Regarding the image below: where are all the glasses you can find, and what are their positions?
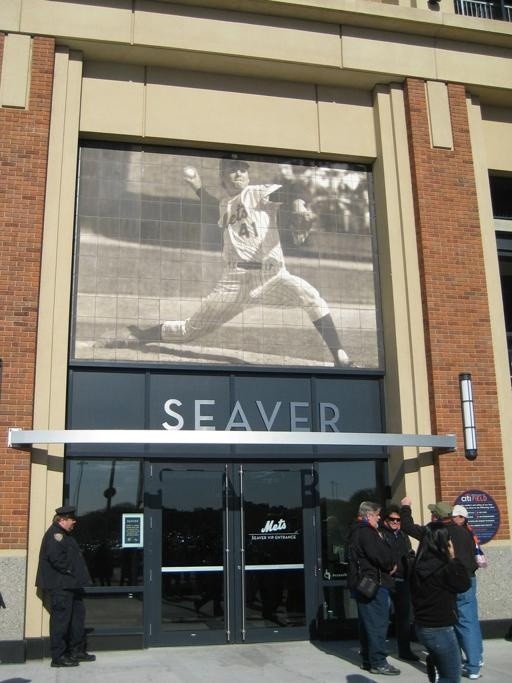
[387,516,401,522]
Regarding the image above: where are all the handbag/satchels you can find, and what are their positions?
[354,560,379,602]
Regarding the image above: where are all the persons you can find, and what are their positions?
[34,505,96,667]
[269,158,371,257]
[126,157,358,367]
[321,495,489,683]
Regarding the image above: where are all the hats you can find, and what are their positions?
[55,506,78,514]
[219,156,249,169]
[427,502,452,519]
[450,504,469,520]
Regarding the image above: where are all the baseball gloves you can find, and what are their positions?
[289,210,317,245]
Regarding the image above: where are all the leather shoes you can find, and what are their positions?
[50,656,79,667]
[400,653,420,662]
[70,651,96,662]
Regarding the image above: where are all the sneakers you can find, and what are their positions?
[360,661,371,670]
[369,662,400,675]
[98,324,140,349]
[461,657,484,667]
[469,665,481,679]
[333,349,359,368]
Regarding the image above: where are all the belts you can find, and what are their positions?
[236,262,267,270]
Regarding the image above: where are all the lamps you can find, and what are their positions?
[459,370,480,460]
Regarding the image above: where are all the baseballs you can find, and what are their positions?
[183,167,196,178]
